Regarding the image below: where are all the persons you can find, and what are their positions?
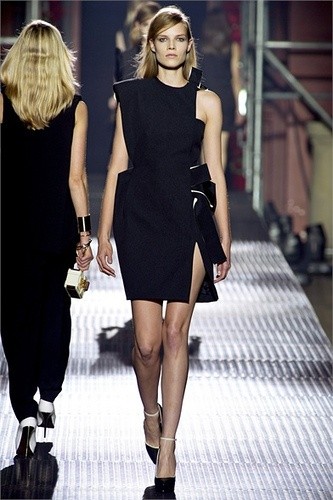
[94,6,231,493]
[110,0,244,181]
[0,20,93,464]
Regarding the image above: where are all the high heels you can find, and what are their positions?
[14,416,39,457]
[144,403,177,496]
[38,399,55,438]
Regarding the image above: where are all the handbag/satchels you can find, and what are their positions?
[63,238,92,299]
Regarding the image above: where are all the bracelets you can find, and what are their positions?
[76,214,92,250]
